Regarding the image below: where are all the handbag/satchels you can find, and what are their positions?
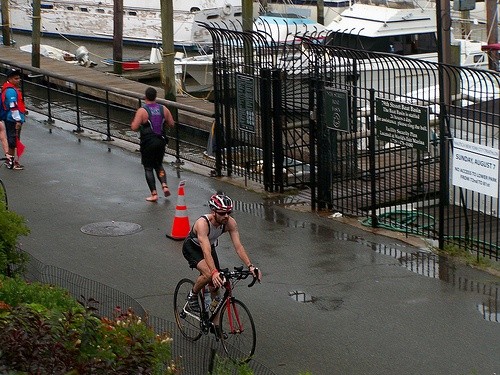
[0,92,9,121]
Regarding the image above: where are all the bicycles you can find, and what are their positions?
[0,156,14,211]
[173,266,261,364]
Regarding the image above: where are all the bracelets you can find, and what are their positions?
[211,269,218,276]
[5,152,10,156]
[248,264,254,269]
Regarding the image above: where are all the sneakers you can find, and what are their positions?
[185,290,201,315]
[210,326,228,340]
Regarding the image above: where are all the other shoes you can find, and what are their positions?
[4,160,24,170]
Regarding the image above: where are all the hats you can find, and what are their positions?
[7,68,20,79]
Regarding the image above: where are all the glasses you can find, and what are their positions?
[213,210,232,216]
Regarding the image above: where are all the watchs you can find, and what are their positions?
[181,192,260,332]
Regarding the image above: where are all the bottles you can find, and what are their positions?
[210,295,220,311]
[204,289,211,311]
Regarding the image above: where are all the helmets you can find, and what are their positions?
[208,193,233,212]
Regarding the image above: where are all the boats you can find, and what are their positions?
[7,0,500,150]
[0,33,16,48]
[101,58,163,72]
[203,146,319,192]
[19,43,98,90]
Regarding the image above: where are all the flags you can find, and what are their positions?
[15,136,25,158]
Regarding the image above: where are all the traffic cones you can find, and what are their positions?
[166,183,191,240]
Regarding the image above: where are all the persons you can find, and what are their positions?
[0,120,11,163]
[5,68,28,170]
[132,87,176,201]
